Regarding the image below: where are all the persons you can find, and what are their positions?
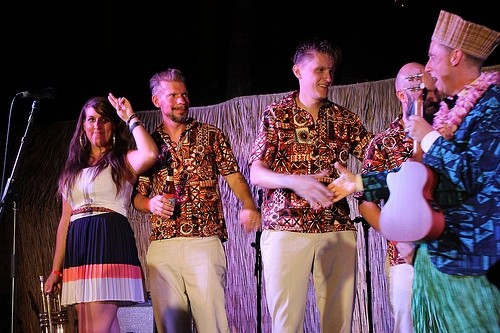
[44,93,158,333]
[131,70,261,333]
[327,10,500,333]
[249,40,376,333]
[357,63,438,333]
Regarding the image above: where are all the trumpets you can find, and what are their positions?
[39,274,53,332]
[52,288,69,333]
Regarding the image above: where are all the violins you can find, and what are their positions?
[377,83,445,242]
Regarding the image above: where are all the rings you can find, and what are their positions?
[251,221,256,224]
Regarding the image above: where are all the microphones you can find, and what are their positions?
[16,87,55,99]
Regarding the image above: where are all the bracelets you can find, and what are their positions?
[127,113,145,133]
[51,270,61,275]
[145,197,153,212]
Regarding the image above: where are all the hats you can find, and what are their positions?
[432,9,499,60]
